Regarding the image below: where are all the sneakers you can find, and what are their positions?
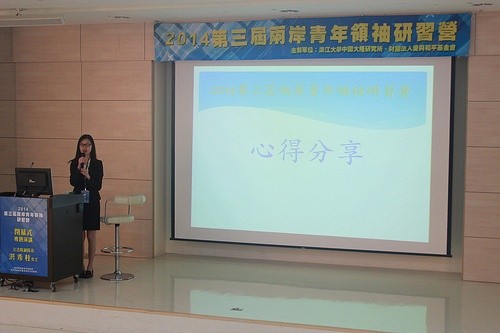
[79,270,86,278]
[85,270,93,278]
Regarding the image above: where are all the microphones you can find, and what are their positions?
[81,153,85,168]
[30,162,34,167]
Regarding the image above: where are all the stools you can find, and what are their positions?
[99,195,146,282]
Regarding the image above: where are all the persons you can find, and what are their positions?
[69,134,104,279]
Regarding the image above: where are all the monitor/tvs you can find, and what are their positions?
[15,167,52,198]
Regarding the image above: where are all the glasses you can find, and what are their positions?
[79,143,92,148]
